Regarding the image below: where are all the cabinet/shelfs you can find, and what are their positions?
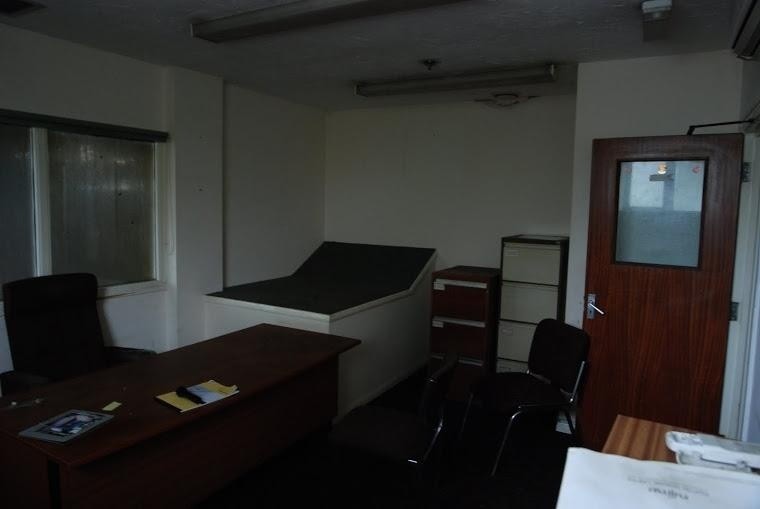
[496,232,567,377]
[430,263,498,377]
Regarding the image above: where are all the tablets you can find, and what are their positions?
[18,407,116,447]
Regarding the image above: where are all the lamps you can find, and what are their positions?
[354,63,558,98]
[188,0,451,46]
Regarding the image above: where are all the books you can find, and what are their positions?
[155,379,241,414]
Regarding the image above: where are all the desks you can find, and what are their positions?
[2,324,366,508]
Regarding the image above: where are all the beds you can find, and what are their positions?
[203,241,442,425]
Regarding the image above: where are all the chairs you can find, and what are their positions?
[457,318,590,475]
[3,272,159,387]
